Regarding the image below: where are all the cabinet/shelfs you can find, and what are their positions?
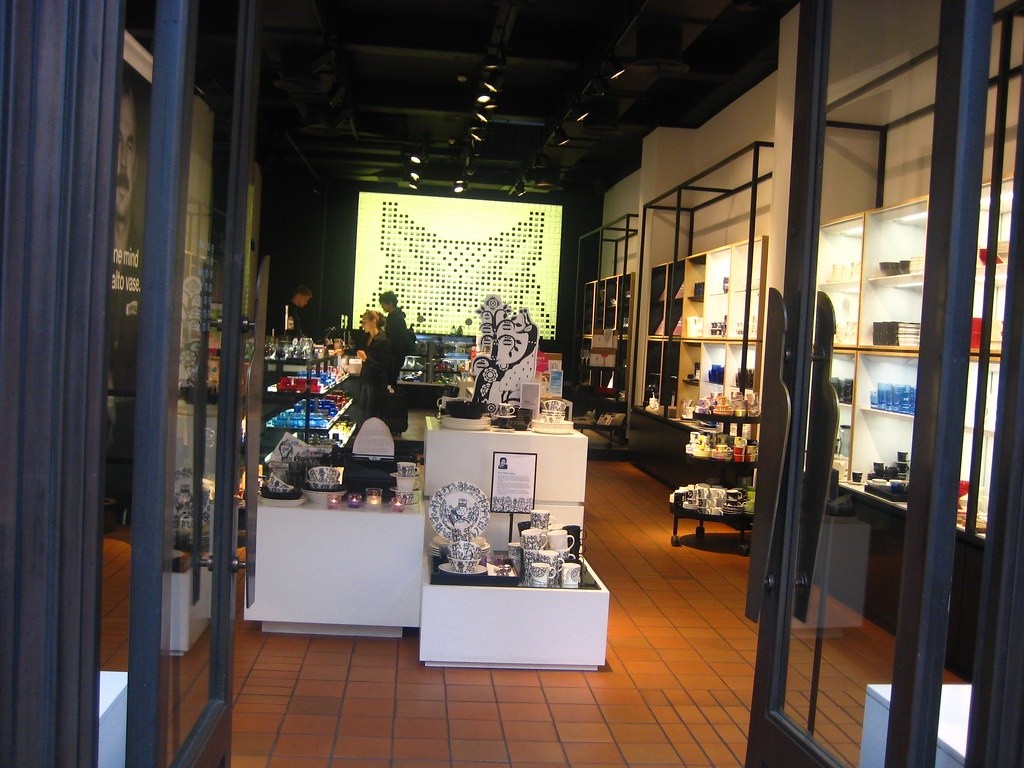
[813,176,1015,538]
[641,231,769,442]
[671,411,762,555]
[581,271,636,399]
[398,335,473,386]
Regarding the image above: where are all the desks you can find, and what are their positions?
[423,416,589,554]
[244,490,424,638]
[264,348,356,450]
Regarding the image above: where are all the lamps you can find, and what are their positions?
[409,43,625,200]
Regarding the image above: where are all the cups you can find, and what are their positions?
[498,403,515,416]
[326,462,421,511]
[820,262,862,484]
[868,382,916,414]
[434,385,461,422]
[645,276,761,427]
[685,431,758,461]
[265,337,353,447]
[668,483,754,515]
[507,508,587,588]
[853,451,909,502]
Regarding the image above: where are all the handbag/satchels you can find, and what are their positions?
[366,355,400,382]
[401,324,418,356]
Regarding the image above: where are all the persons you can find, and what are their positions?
[379,291,407,385]
[284,285,312,337]
[114,87,139,338]
[357,311,387,418]
[498,457,507,469]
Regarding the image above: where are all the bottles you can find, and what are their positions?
[450,325,456,336]
[456,326,463,336]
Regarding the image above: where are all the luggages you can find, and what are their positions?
[383,383,409,437]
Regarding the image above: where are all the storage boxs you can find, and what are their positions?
[419,545,610,670]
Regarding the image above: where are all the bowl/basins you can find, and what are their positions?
[541,400,566,422]
[510,408,532,430]
[447,542,482,559]
[267,487,294,493]
[447,554,481,572]
[300,467,347,502]
[268,473,291,487]
[445,400,487,418]
[871,254,925,349]
[970,241,1011,353]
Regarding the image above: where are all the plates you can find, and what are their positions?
[442,414,492,431]
[427,482,491,557]
[257,487,306,506]
[495,415,516,418]
[531,419,574,435]
[438,563,488,575]
